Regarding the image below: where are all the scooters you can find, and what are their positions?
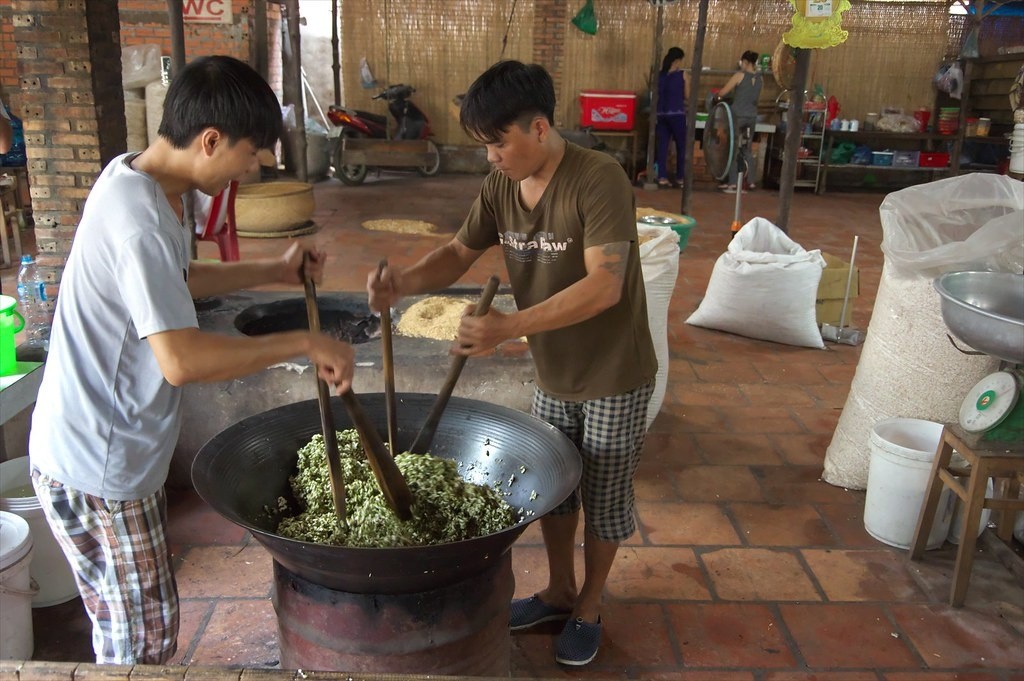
[328,83,444,185]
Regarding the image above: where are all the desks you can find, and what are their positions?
[767,90,828,194]
[588,131,639,185]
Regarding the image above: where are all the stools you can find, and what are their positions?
[0,186,23,265]
[912,422,1024,607]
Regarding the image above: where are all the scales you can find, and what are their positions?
[933,270,1024,459]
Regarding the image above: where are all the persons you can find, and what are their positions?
[29,56,353,665]
[715,51,764,192]
[0,98,12,154]
[656,47,690,187]
[366,60,658,666]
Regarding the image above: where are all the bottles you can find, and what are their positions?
[865,111,879,131]
[965,117,992,137]
[913,106,932,134]
[17,256,51,350]
[829,117,859,131]
[0,105,27,166]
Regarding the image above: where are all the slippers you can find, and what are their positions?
[510,593,570,630]
[556,612,602,666]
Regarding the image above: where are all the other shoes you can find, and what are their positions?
[719,184,756,193]
[659,179,673,188]
[677,180,684,188]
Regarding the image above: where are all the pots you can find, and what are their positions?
[192,393,583,595]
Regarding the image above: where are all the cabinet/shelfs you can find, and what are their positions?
[820,127,961,194]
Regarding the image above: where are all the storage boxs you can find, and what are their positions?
[890,149,921,167]
[580,90,638,132]
[920,152,950,167]
[872,152,892,166]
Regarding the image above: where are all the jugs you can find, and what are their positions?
[824,95,842,127]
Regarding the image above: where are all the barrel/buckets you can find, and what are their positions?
[1009,124,1024,174]
[0,456,80,608]
[0,511,40,662]
[865,418,960,549]
[1,296,25,376]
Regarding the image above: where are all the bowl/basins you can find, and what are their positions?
[640,215,676,225]
[934,269,1024,365]
[939,108,959,134]
[654,214,696,252]
